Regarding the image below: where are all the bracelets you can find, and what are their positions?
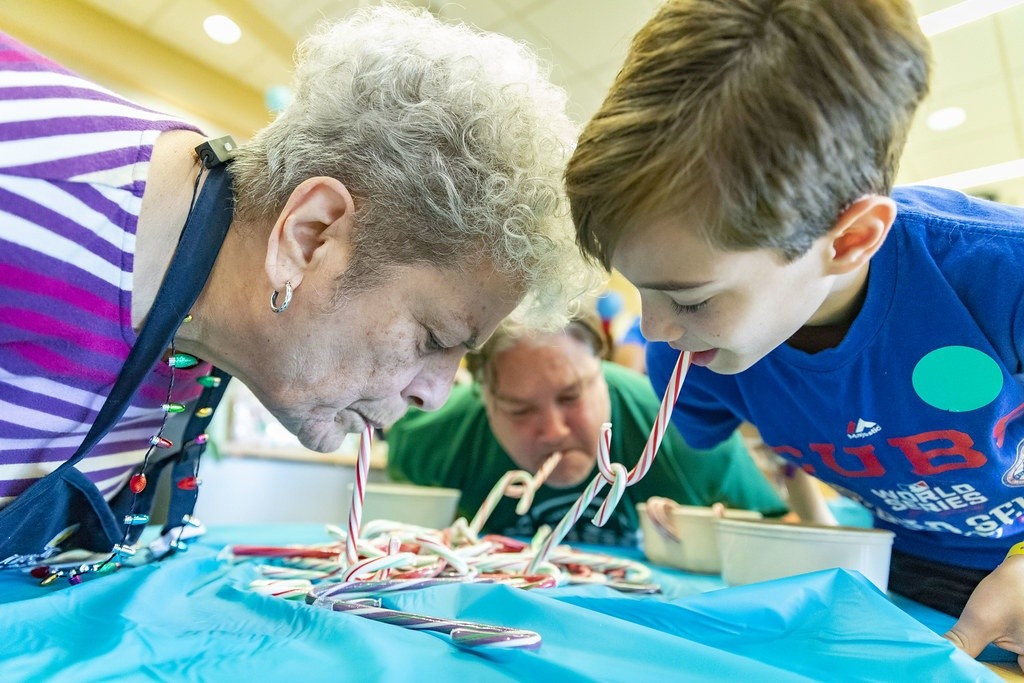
[1006,542,1024,557]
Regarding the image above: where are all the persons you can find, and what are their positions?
[385,304,789,549]
[0,7,607,553]
[564,0,1024,678]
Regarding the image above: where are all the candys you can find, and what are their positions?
[233,350,692,652]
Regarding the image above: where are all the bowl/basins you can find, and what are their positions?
[637,503,762,575]
[711,517,898,595]
[346,481,459,531]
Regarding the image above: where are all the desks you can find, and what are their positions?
[192,445,387,520]
[0,520,1024,683]
